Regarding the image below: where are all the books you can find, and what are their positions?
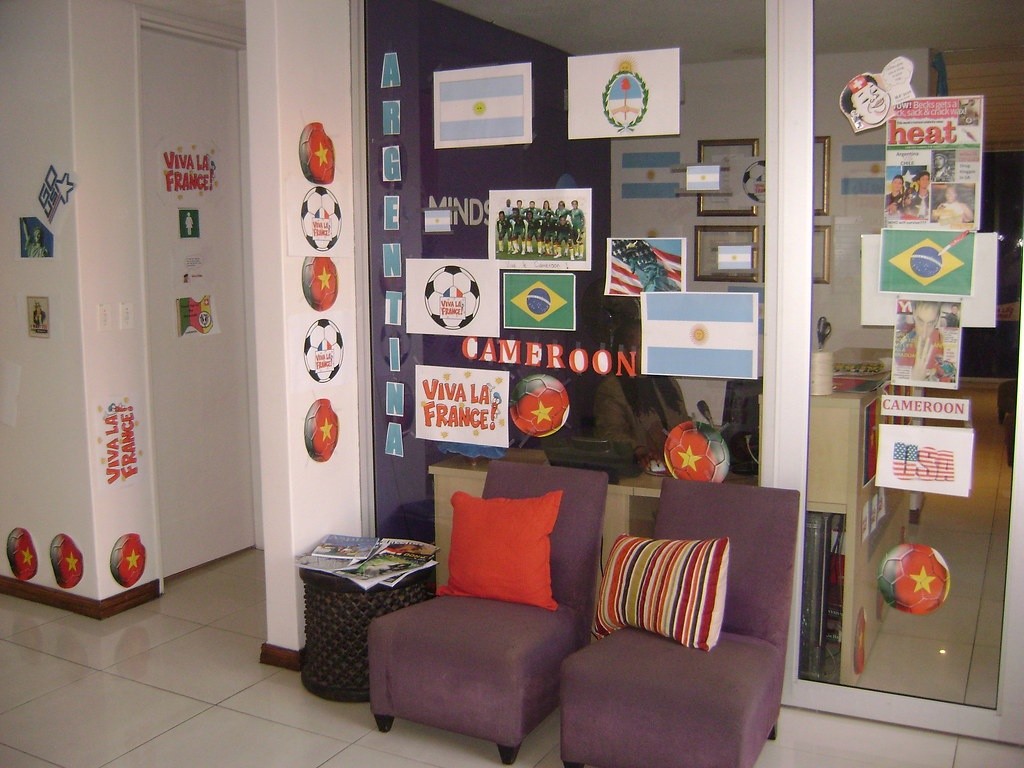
[298,534,440,589]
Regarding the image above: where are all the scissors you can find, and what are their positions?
[816,315,832,352]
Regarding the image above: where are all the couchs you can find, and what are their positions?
[368,462,609,765]
[559,479,801,768]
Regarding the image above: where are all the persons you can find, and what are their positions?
[946,305,959,328]
[886,174,903,217]
[895,300,944,382]
[497,199,586,260]
[905,171,930,220]
[934,185,974,224]
[592,320,688,476]
[627,251,681,292]
[933,150,952,182]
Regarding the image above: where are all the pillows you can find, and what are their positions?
[593,531,735,652]
[437,488,566,609]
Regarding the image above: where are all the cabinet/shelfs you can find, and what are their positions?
[757,345,929,685]
[428,447,758,641]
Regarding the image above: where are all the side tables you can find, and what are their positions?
[298,560,429,705]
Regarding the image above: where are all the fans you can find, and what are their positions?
[581,278,641,351]
